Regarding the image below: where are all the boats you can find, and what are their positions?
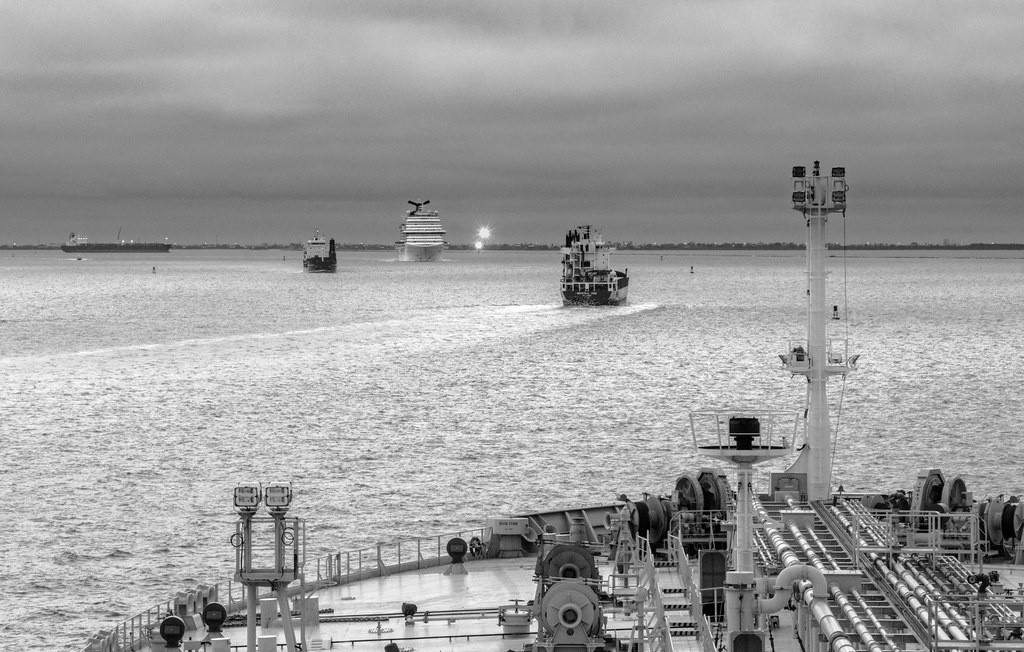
[558,223,629,307]
[301,229,337,274]
[395,198,449,262]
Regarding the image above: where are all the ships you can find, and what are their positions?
[59,227,170,254]
